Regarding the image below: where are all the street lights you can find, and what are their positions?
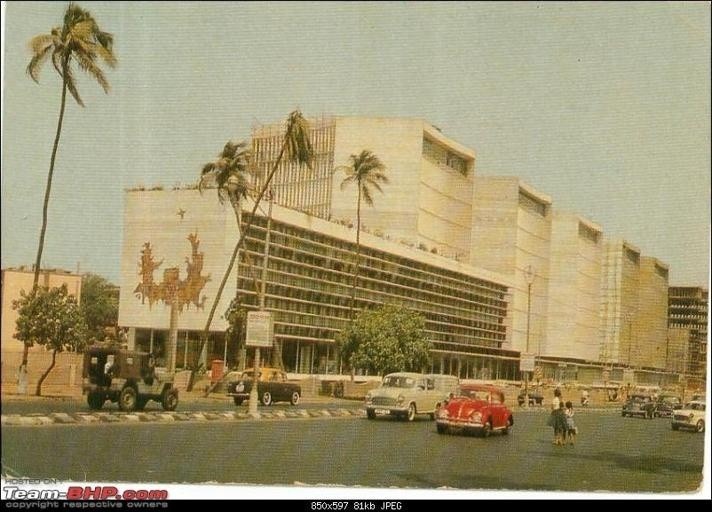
[522,264,536,409]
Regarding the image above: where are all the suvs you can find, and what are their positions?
[82,347,179,412]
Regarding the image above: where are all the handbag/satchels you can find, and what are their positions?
[573,426,577,434]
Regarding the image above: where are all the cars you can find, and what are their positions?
[518,391,545,408]
[364,372,459,422]
[621,394,706,434]
[436,383,514,438]
[226,367,301,406]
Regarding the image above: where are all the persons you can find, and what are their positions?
[547,389,579,447]
